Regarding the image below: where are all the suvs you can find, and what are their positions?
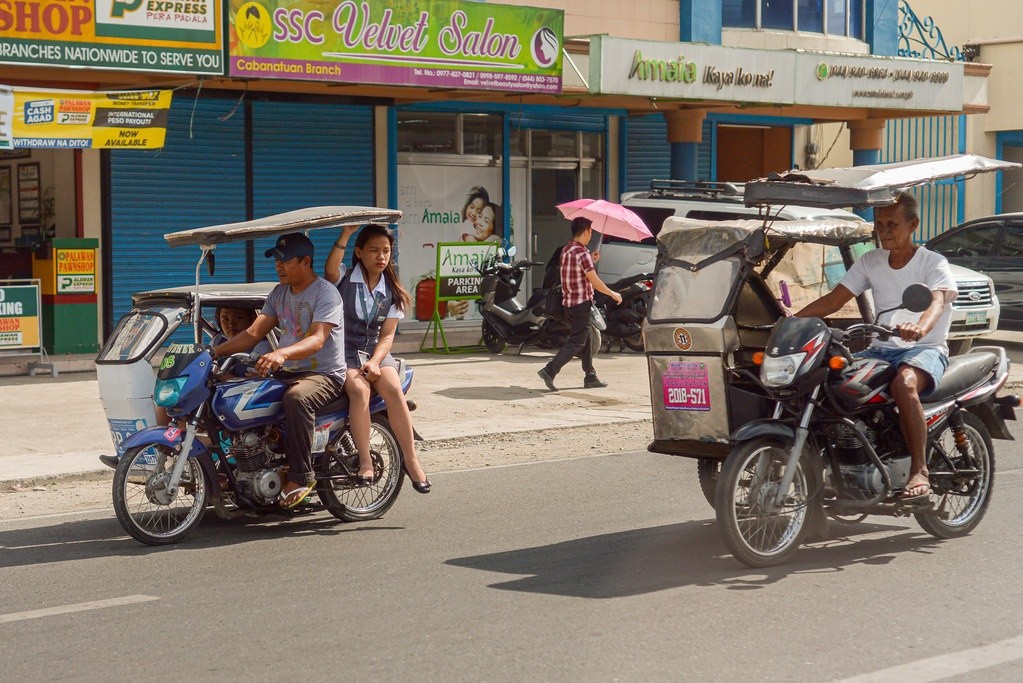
[593,177,1001,359]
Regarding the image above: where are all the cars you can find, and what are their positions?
[918,211,1023,335]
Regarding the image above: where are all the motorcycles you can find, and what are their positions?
[641,152,1023,569]
[542,245,654,353]
[468,236,609,359]
[97,205,413,546]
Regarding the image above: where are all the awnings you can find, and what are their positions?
[164,205,404,247]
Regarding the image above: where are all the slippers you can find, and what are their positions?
[900,482,932,502]
[279,478,318,510]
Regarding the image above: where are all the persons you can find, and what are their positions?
[99,307,271,470]
[537,217,622,392]
[210,233,347,509]
[325,225,432,493]
[447,185,501,317]
[535,246,566,348]
[788,192,960,504]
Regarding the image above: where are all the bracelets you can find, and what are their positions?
[333,241,347,250]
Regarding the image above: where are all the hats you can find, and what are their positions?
[265,232,315,260]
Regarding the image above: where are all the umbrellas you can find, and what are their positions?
[555,198,655,252]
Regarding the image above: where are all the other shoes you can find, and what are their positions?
[538,369,558,391]
[584,380,608,388]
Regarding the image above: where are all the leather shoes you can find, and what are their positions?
[401,459,432,494]
[356,467,375,484]
